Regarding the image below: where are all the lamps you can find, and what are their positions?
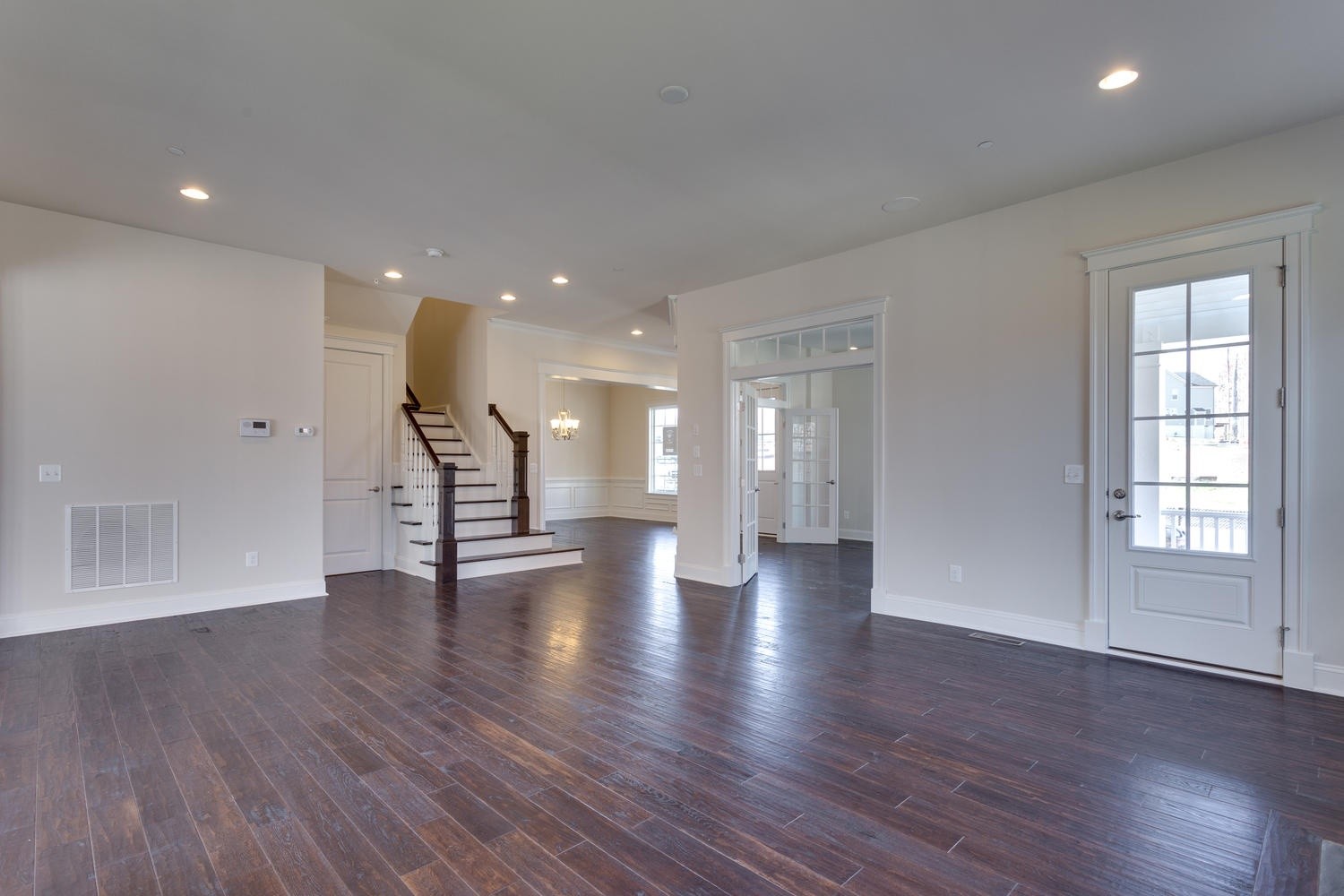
[548,378,579,441]
